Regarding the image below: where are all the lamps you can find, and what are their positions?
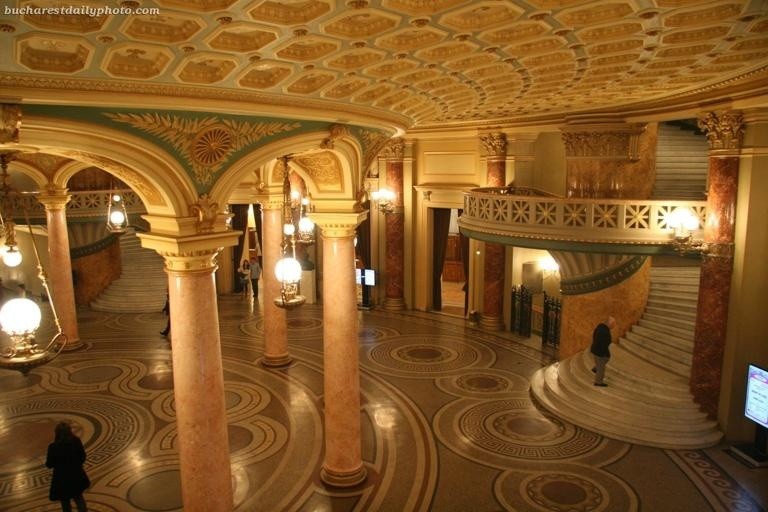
[668,205,700,242]
[368,186,396,217]
[282,182,317,243]
[105,178,131,234]
[1,212,25,266]
[272,211,308,309]
[0,245,67,369]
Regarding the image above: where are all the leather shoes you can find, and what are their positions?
[592,368,596,373]
[160,330,168,336]
[594,382,608,387]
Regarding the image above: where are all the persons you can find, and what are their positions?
[238,258,250,294]
[159,294,170,336]
[588,316,616,387]
[250,256,260,297]
[45,422,92,511]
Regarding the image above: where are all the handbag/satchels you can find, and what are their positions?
[239,272,247,278]
